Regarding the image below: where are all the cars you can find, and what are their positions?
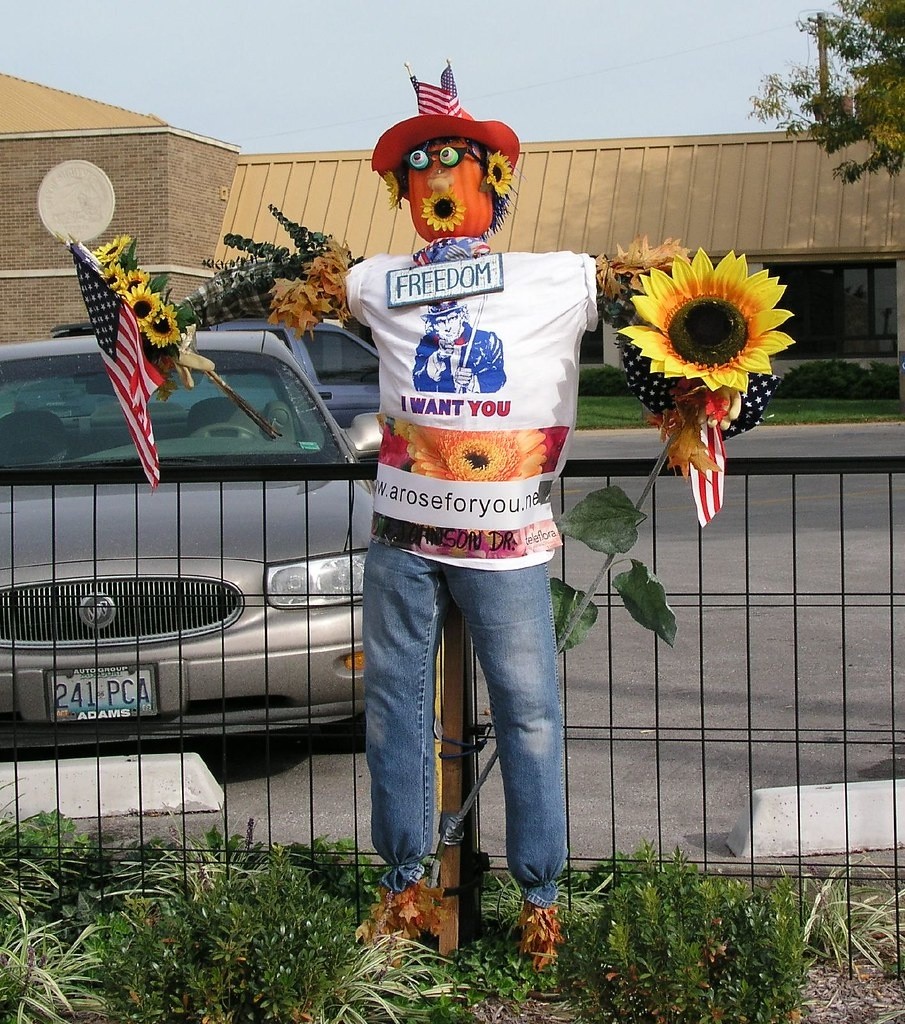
[198,317,381,461]
[13,377,96,423]
[1,327,376,751]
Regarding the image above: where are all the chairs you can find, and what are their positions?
[0,410,68,466]
[186,396,262,437]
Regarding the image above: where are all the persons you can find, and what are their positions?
[49,59,797,972]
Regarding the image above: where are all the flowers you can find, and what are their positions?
[384,169,403,216]
[613,249,796,484]
[421,188,466,234]
[482,146,511,200]
[369,417,566,558]
[88,235,186,356]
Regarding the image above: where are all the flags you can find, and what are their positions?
[64,239,165,491]
[405,65,475,121]
[621,335,784,529]
